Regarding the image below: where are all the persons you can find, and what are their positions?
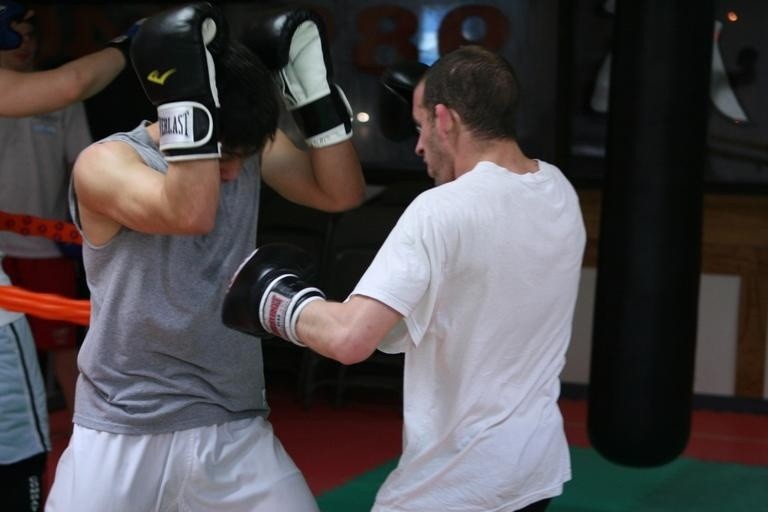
[2,18,146,512]
[8,5,92,434]
[219,44,591,512]
[40,2,366,512]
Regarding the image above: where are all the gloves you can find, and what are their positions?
[255,6,357,149]
[219,238,328,348]
[126,0,234,162]
